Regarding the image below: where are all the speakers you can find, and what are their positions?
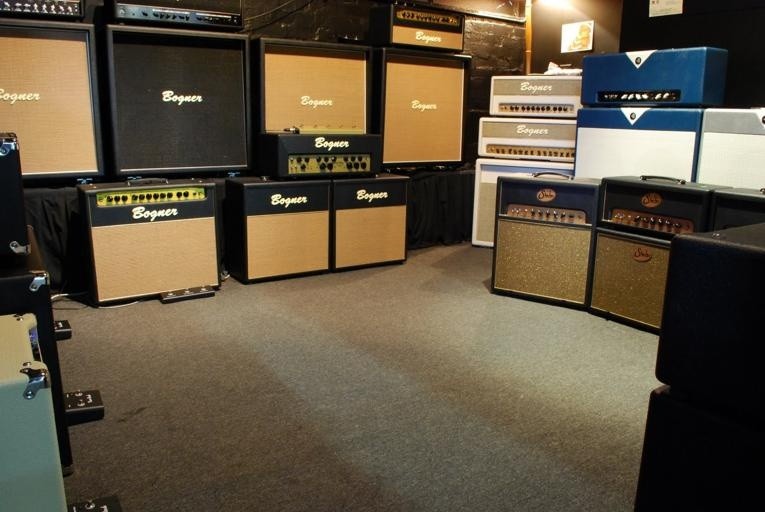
[378,50,471,165]
[633,221,764,510]
[106,23,252,175]
[372,2,466,54]
[225,178,330,283]
[257,37,371,134]
[472,46,765,337]
[332,173,413,273]
[0,18,104,179]
[77,178,220,302]
